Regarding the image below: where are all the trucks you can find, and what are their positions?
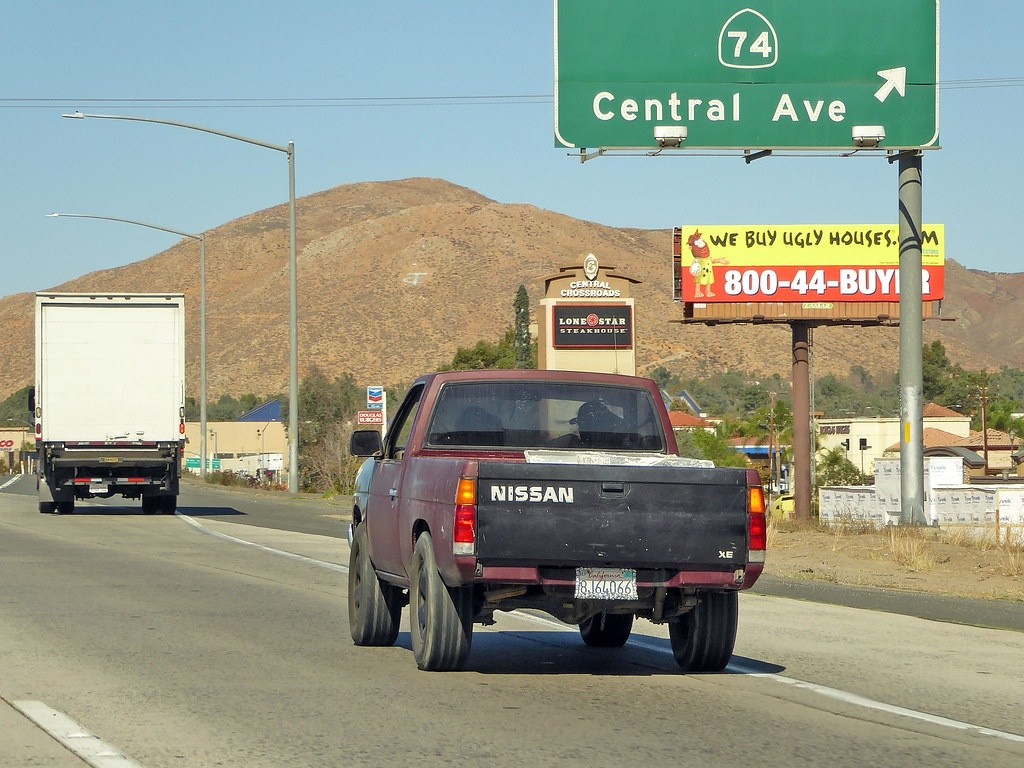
[27,290,188,516]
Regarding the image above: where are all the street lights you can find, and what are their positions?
[62,111,298,494]
[1010,430,1016,475]
[45,213,206,481]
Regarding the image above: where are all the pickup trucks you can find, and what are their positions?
[346,369,767,675]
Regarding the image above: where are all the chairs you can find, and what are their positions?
[578,413,629,449]
[451,414,505,446]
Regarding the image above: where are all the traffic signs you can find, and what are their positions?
[552,0,945,151]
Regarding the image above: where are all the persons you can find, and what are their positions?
[455,406,494,434]
[569,400,613,450]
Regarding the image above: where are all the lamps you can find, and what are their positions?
[654,126,688,147]
[851,126,885,147]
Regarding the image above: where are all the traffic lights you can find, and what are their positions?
[268,471,273,480]
[256,468,260,479]
[841,439,849,450]
